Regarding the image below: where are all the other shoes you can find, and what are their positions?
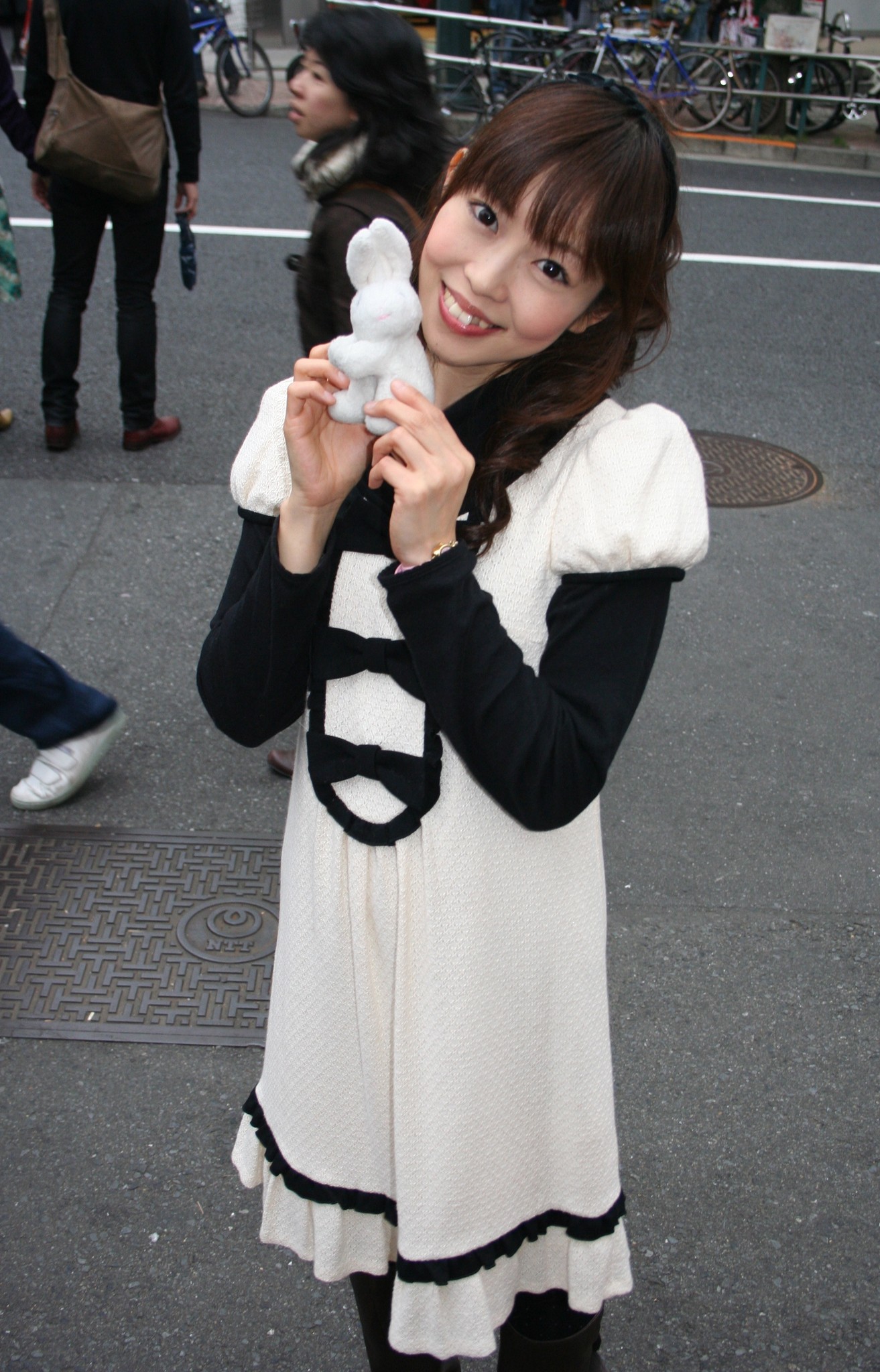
[268,748,296,779]
[0,408,12,428]
[196,87,206,99]
[227,73,239,95]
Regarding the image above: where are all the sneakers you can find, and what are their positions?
[9,708,127,810]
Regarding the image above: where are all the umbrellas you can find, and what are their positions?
[174,210,197,290]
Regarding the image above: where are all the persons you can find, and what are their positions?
[0,620,130,811]
[196,80,708,1372]
[0,0,240,453]
[268,5,451,780]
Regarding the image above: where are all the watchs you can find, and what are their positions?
[393,541,458,574]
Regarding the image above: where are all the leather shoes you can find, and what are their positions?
[123,416,179,450]
[46,418,80,451]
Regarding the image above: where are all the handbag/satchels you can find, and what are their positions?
[718,0,757,66]
[34,73,166,195]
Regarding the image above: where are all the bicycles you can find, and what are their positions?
[191,0,274,118]
[424,0,880,151]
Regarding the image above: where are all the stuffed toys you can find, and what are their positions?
[326,219,435,436]
